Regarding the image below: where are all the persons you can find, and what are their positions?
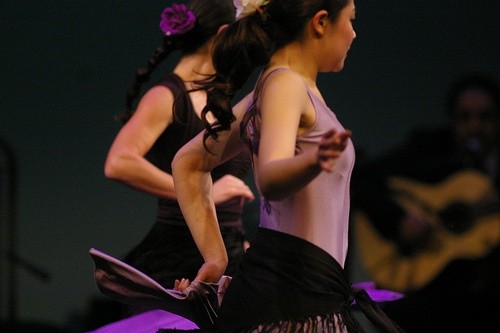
[105,1,499,333]
